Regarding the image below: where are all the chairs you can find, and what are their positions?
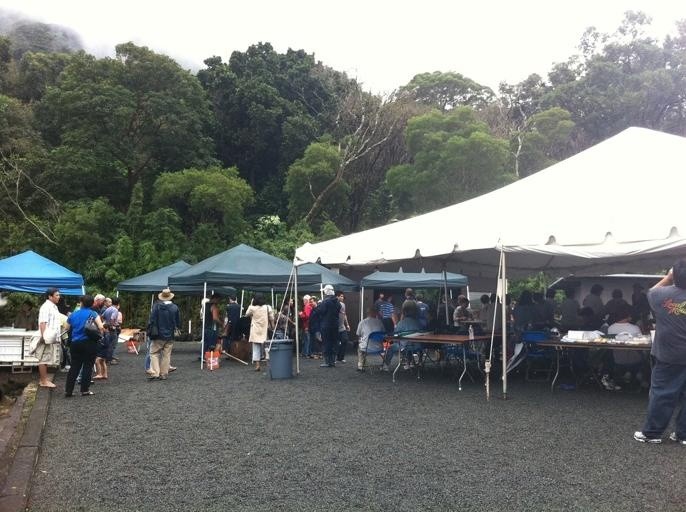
[358,319,656,392]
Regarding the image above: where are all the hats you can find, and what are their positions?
[324,285,335,296]
[158,288,175,301]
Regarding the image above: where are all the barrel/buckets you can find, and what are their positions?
[127,340,138,354]
[204,350,220,371]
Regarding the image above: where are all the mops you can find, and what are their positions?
[207,344,215,371]
[213,343,249,365]
[129,337,139,355]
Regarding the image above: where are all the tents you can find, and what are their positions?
[0,244,468,369]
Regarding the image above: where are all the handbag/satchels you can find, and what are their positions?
[85,317,105,344]
[149,320,159,340]
[173,327,182,337]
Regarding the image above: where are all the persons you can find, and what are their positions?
[14,255,685,396]
[633,259,686,446]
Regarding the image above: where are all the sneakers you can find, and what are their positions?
[669,431,686,445]
[147,365,176,380]
[633,431,662,443]
[601,374,622,390]
